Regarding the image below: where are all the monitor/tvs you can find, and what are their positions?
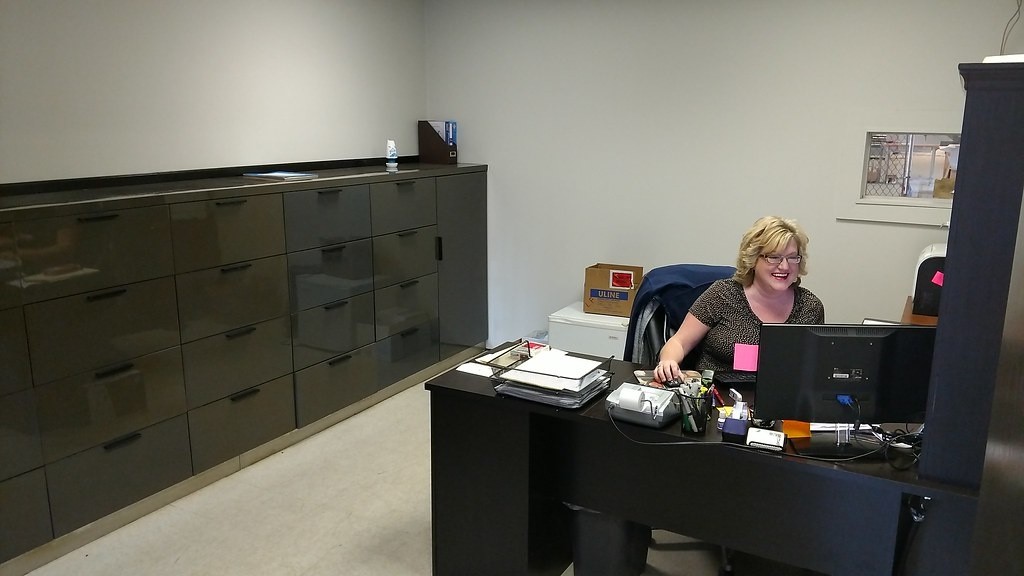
[752,323,936,460]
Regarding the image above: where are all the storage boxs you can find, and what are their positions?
[583,262,644,317]
[547,302,629,360]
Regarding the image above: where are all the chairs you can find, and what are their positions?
[622,264,737,365]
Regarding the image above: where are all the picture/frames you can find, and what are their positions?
[835,107,964,228]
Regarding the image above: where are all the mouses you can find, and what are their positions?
[663,373,680,387]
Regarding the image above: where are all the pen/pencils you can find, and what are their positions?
[713,389,725,405]
[678,381,714,435]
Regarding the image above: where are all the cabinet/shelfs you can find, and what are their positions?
[0,162,488,576]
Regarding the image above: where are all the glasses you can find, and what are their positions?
[758,254,802,264]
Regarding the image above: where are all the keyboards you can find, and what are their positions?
[714,372,757,385]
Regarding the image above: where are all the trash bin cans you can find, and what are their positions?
[566,503,653,576]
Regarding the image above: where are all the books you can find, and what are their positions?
[244,170,320,181]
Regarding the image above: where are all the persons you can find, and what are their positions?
[653,218,825,383]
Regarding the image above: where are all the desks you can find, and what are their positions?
[425,341,979,576]
